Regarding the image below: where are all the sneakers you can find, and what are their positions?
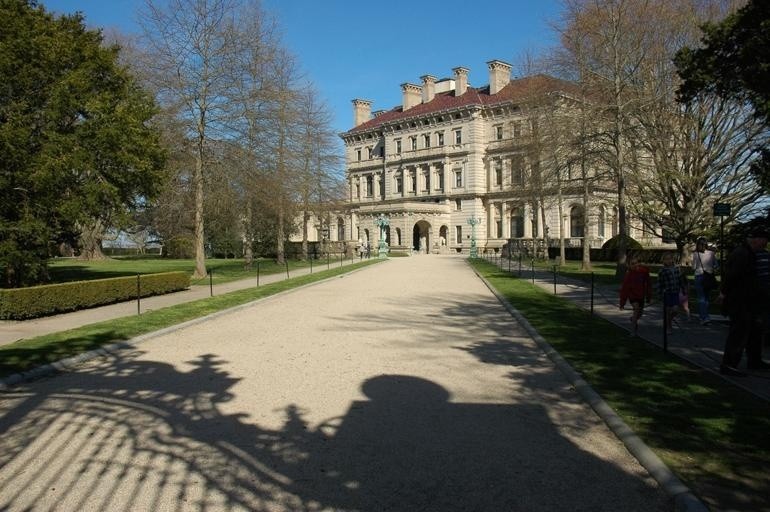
[747,360,770,372]
[700,319,713,327]
[719,364,747,377]
[630,317,639,329]
[632,327,640,338]
[666,328,673,335]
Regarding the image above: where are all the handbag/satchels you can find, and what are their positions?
[701,269,718,291]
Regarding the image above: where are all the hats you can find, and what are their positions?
[747,229,770,238]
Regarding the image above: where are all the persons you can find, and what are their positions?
[619,256,654,336]
[692,236,719,323]
[716,227,769,376]
[757,248,769,364]
[359,243,364,259]
[365,243,370,258]
[679,283,694,322]
[657,253,690,334]
